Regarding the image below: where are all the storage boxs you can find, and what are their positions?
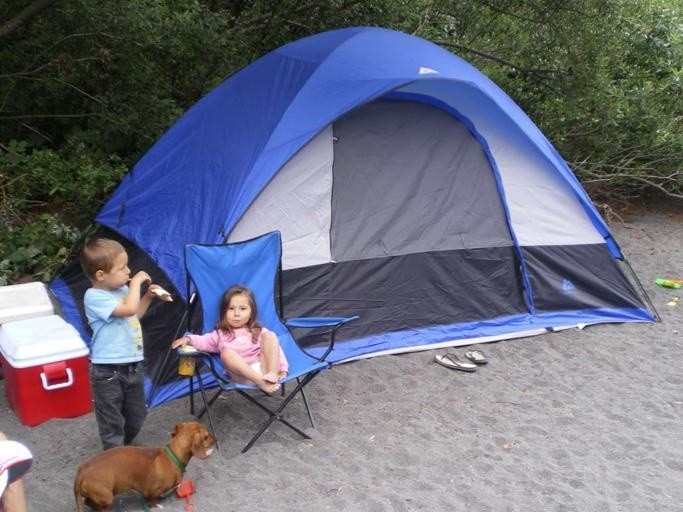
[1,281,94,429]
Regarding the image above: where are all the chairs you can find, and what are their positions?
[170,230,361,456]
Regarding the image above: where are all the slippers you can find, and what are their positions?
[434,353,476,371]
[465,348,487,364]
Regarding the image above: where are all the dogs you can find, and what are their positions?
[73,419,217,511]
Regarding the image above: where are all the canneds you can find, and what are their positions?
[178,345,196,376]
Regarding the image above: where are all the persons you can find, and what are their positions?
[81,236,174,451]
[0,429,35,512]
[171,284,290,395]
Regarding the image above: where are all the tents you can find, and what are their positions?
[46,25,663,413]
